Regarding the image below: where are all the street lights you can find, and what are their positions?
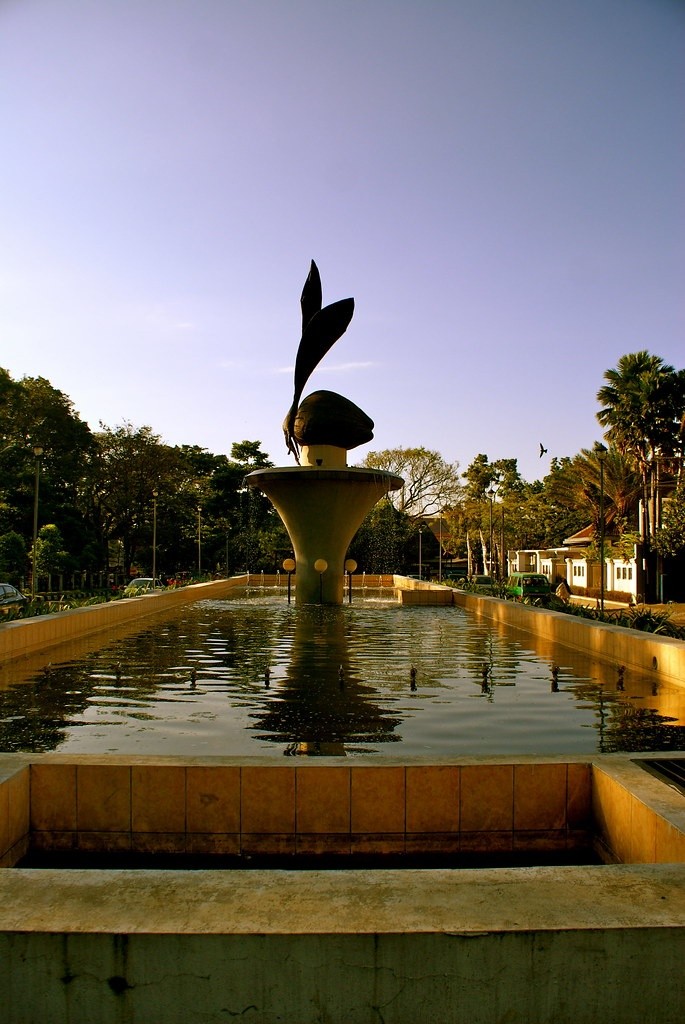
[488,487,496,578]
[416,521,428,579]
[197,505,203,577]
[152,487,160,589]
[31,439,46,599]
[595,442,608,614]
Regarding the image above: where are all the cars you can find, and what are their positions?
[0,583,29,618]
[408,573,467,585]
[471,575,496,590]
[124,578,165,596]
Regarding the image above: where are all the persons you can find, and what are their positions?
[559,578,573,604]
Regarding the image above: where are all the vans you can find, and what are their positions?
[506,573,551,601]
